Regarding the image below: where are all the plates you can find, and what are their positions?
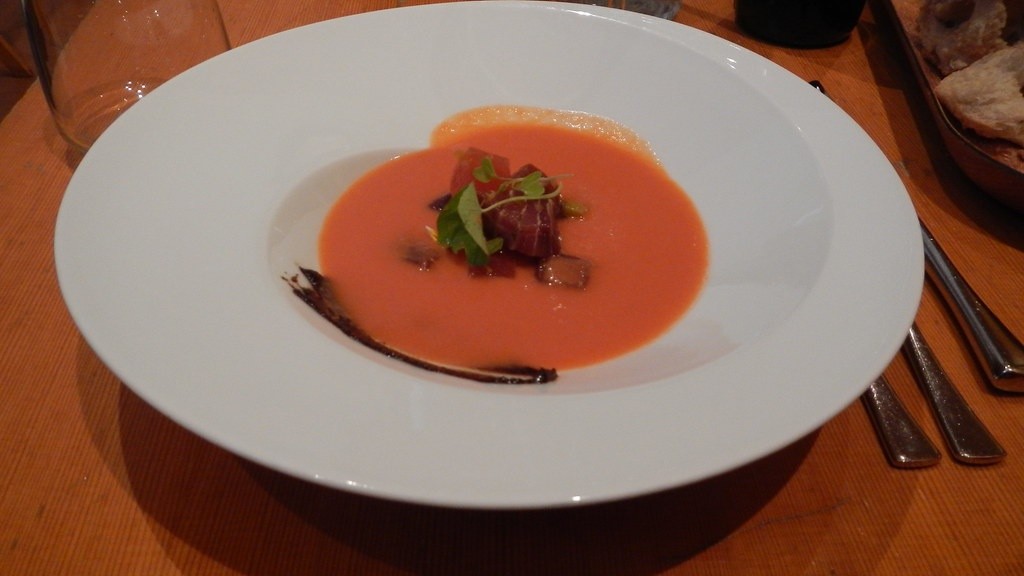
[869,0,1024,210]
[54,0,924,510]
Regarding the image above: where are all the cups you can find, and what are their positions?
[732,0,866,51]
[22,0,234,155]
